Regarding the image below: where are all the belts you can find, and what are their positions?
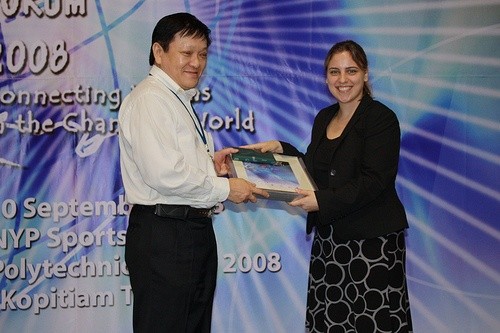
[135,203,214,218]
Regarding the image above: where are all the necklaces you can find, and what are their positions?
[169,88,210,153]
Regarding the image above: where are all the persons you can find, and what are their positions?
[238,39,414,333]
[116,12,270,333]
